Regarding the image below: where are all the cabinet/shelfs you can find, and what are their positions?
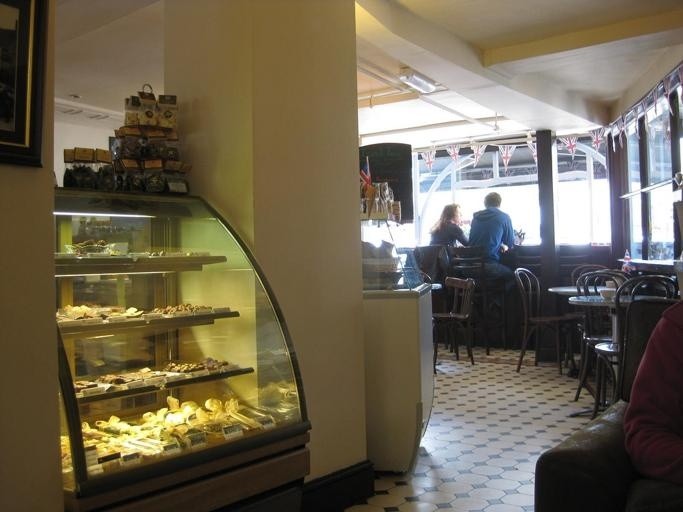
[54,188,311,510]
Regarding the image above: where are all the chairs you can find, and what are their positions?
[514,265,678,420]
[534,299,682,510]
[416,245,487,373]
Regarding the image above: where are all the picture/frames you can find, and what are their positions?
[1,2,49,168]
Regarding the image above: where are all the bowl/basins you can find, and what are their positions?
[599,289,616,300]
[605,280,615,288]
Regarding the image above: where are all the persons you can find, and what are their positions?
[467,197,517,313]
[430,205,471,279]
[622,300,683,512]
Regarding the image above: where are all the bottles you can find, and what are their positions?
[624,248,630,261]
